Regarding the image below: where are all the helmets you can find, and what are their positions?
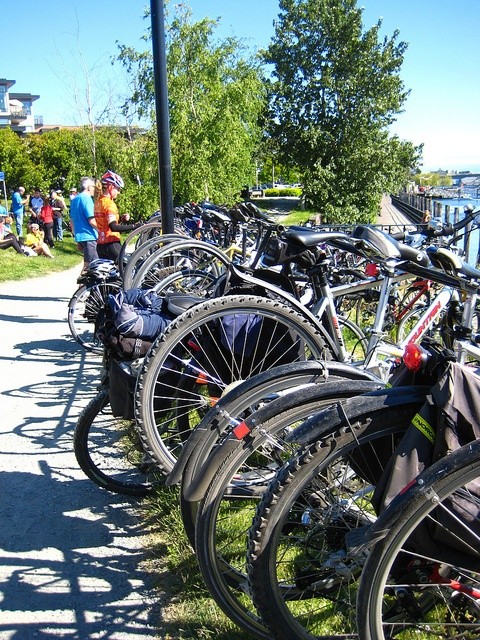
[101,170,125,189]
[81,258,120,280]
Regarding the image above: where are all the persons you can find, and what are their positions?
[69,177,99,267]
[422,209,430,229]
[69,187,77,201]
[57,190,65,198]
[50,191,68,241]
[29,190,43,223]
[0,216,24,254]
[49,189,56,195]
[39,196,55,248]
[94,170,143,260]
[24,223,55,258]
[0,200,7,214]
[11,186,30,234]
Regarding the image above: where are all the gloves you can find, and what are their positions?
[134,220,144,229]
